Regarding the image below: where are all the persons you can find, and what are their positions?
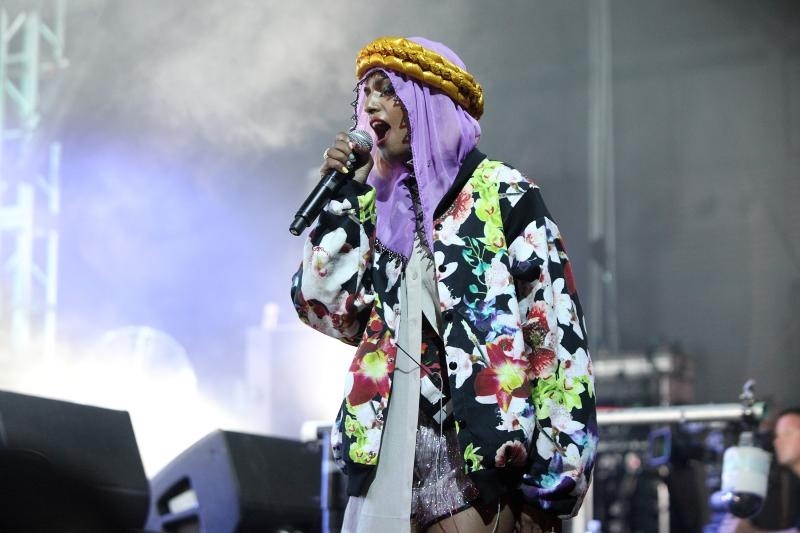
[289,37,599,533]
[717,408,800,533]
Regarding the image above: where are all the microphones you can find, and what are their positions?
[288,129,374,237]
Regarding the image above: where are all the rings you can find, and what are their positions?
[323,148,329,159]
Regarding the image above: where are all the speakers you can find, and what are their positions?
[0,390,151,533]
[144,426,320,533]
